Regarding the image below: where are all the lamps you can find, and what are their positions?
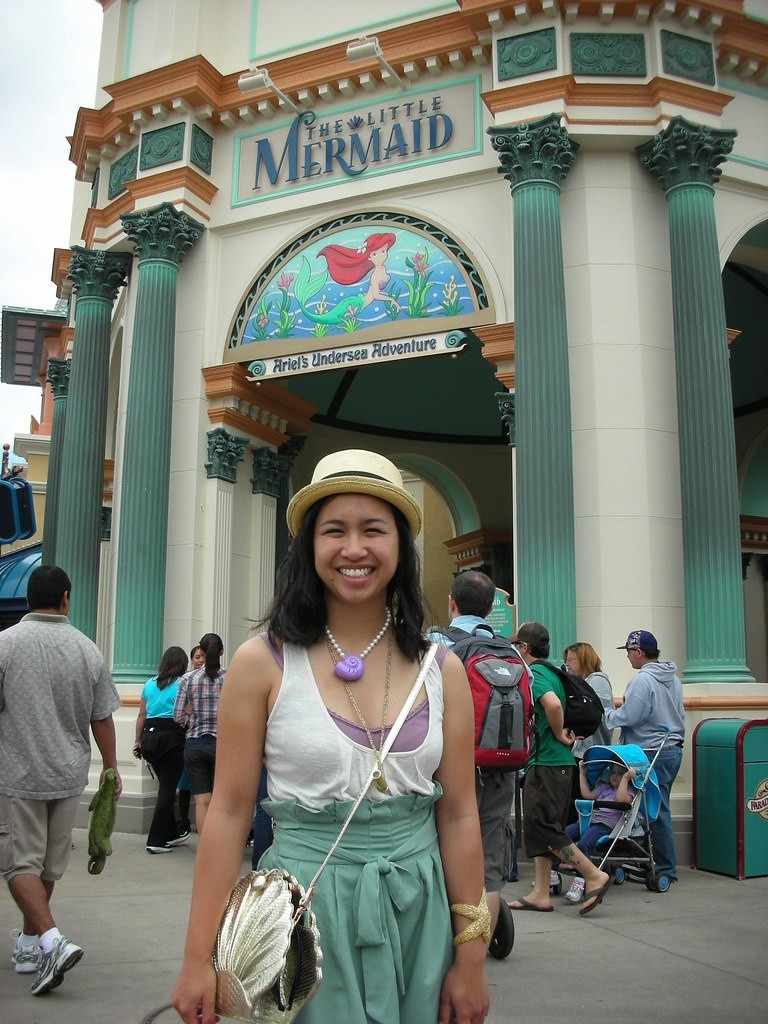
[238,68,302,118]
[345,34,408,93]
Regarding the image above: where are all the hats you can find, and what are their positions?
[286,449,422,540]
[618,630,658,650]
[509,622,550,646]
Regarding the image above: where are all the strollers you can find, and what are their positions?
[552,723,673,896]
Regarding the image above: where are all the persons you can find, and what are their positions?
[132,645,189,855]
[0,565,123,993]
[601,629,686,884]
[171,450,490,1024]
[246,762,274,871]
[563,642,614,823]
[177,646,205,834]
[173,632,227,836]
[532,756,636,902]
[428,570,533,941]
[508,621,616,914]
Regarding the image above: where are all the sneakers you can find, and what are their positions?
[532,869,560,887]
[564,877,586,901]
[10,928,43,972]
[165,830,190,847]
[146,843,172,853]
[31,935,84,994]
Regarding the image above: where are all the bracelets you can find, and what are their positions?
[451,887,492,945]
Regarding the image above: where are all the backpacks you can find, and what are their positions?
[528,660,605,738]
[429,623,539,772]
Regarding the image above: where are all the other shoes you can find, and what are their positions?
[628,870,647,882]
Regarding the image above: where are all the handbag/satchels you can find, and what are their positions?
[210,866,323,1024]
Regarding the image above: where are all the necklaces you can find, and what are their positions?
[324,604,392,681]
[325,625,392,792]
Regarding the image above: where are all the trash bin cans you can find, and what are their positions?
[693,717,768,880]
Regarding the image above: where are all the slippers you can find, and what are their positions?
[579,875,616,915]
[509,898,553,912]
